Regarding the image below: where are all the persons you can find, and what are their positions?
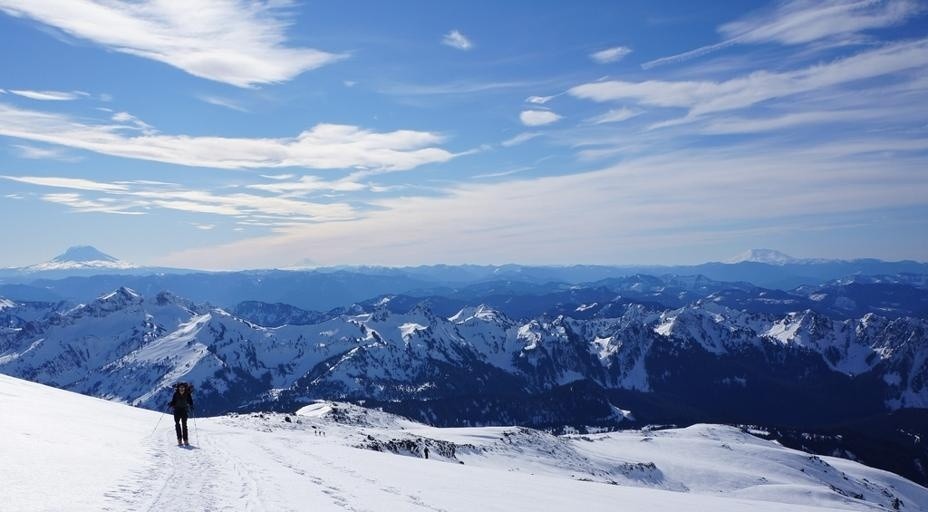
[168,382,195,446]
